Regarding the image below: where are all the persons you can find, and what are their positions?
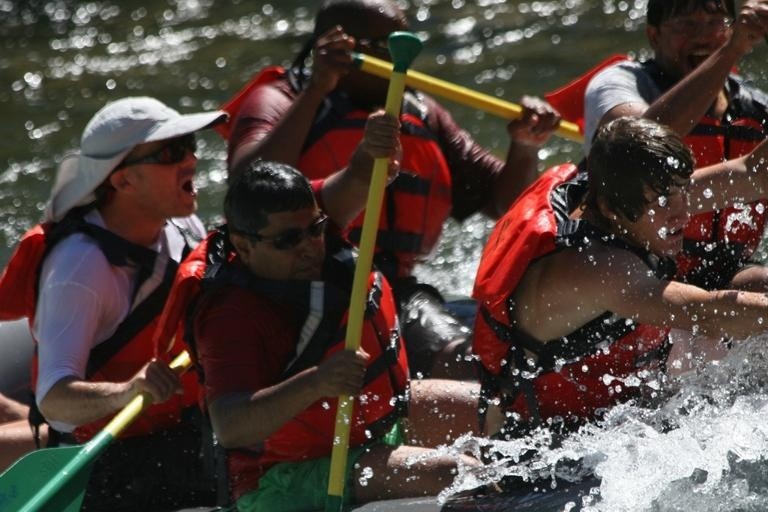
[189,109,509,511]
[0,388,57,475]
[30,93,230,509]
[469,113,768,431]
[223,0,563,382]
[580,1,767,289]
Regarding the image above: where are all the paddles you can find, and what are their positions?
[0,347,191,512]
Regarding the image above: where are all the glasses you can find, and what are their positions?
[113,132,196,170]
[227,210,329,250]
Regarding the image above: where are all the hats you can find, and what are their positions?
[79,95,231,157]
